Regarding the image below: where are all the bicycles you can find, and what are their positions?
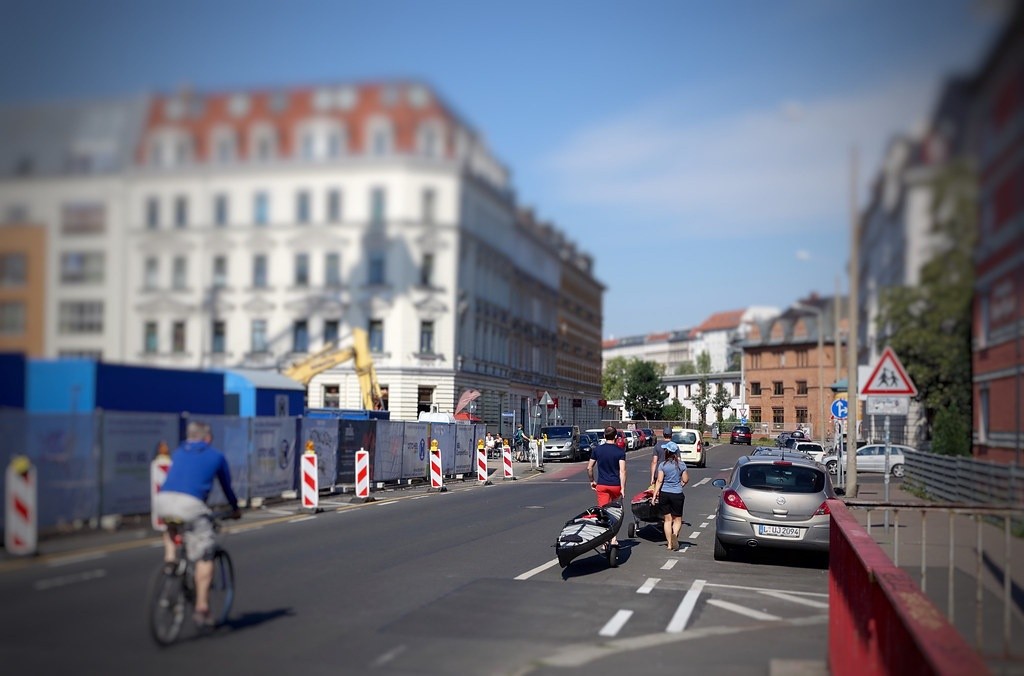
[151,509,243,648]
[511,439,534,461]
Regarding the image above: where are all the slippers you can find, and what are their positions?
[667,534,679,551]
[602,540,621,550]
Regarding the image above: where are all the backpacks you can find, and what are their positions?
[513,430,520,439]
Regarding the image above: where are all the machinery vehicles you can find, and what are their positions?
[286,328,385,411]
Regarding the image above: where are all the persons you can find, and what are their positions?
[485,432,495,460]
[514,423,531,463]
[494,433,503,459]
[652,440,689,552]
[588,425,627,551]
[159,420,242,625]
[651,427,681,488]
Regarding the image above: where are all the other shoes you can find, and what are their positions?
[514,457,520,462]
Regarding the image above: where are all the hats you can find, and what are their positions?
[663,428,672,435]
[661,441,678,452]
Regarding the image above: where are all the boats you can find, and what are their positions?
[631,483,670,525]
[555,495,625,567]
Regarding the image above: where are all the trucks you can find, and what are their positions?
[825,495,1024,675]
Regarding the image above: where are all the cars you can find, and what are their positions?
[710,455,848,560]
[821,445,919,477]
[537,425,826,467]
